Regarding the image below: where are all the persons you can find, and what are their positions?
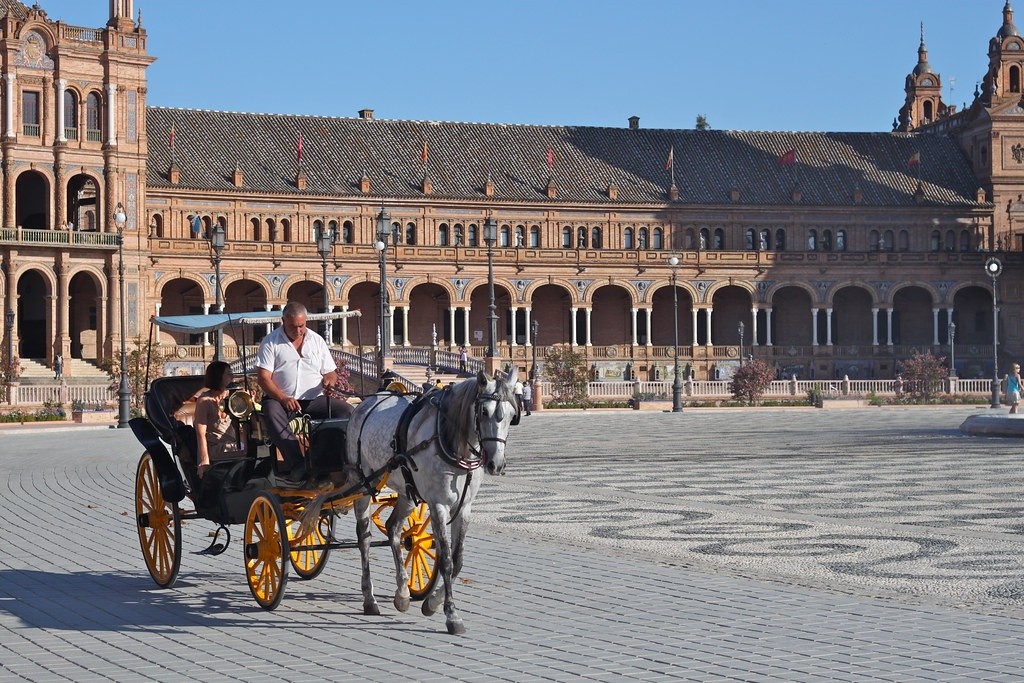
[61,220,73,231]
[54,352,64,379]
[1007,363,1024,414]
[255,301,355,482]
[522,382,532,416]
[435,379,444,388]
[514,379,523,411]
[184,362,266,478]
[459,345,467,372]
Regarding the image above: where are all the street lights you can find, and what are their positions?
[736,321,746,368]
[111,203,132,428]
[664,252,684,411]
[482,214,501,381]
[373,199,395,372]
[985,256,1003,408]
[530,319,539,379]
[210,219,226,362]
[316,226,332,348]
[4,308,15,382]
[947,321,956,378]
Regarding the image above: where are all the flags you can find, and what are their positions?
[170,126,174,145]
[777,149,795,166]
[907,153,920,167]
[547,147,553,172]
[666,149,673,170]
[298,135,302,161]
[421,142,428,161]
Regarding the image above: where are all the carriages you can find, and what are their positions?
[134,308,519,634]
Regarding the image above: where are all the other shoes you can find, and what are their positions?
[289,466,306,482]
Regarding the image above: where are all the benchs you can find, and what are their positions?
[146,372,259,467]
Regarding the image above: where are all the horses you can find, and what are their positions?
[345,369,518,633]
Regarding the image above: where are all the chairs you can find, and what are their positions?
[258,385,357,474]
